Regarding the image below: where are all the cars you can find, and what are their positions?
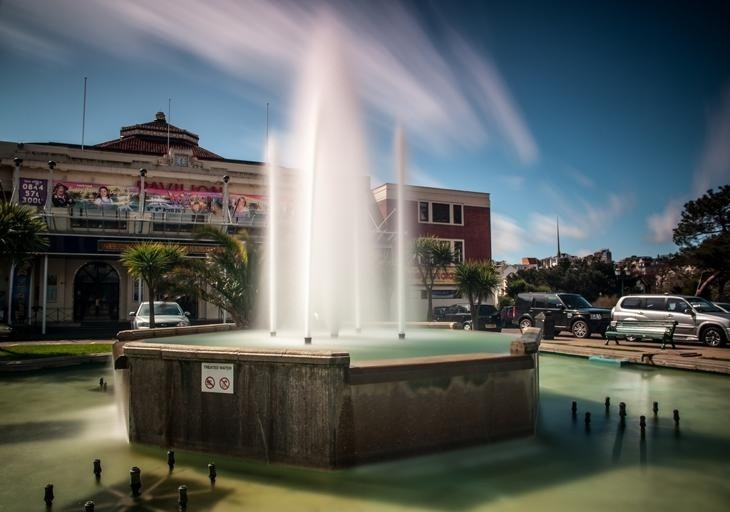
[442,303,503,332]
[128,300,191,330]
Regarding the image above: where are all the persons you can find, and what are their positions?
[189,197,208,213]
[93,186,116,207]
[209,201,229,223]
[121,189,143,213]
[50,181,78,208]
[230,195,250,219]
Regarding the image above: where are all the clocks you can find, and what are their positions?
[169,148,193,169]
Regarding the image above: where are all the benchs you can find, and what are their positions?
[605,319,678,349]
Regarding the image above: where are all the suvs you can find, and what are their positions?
[609,293,730,348]
[514,291,613,338]
